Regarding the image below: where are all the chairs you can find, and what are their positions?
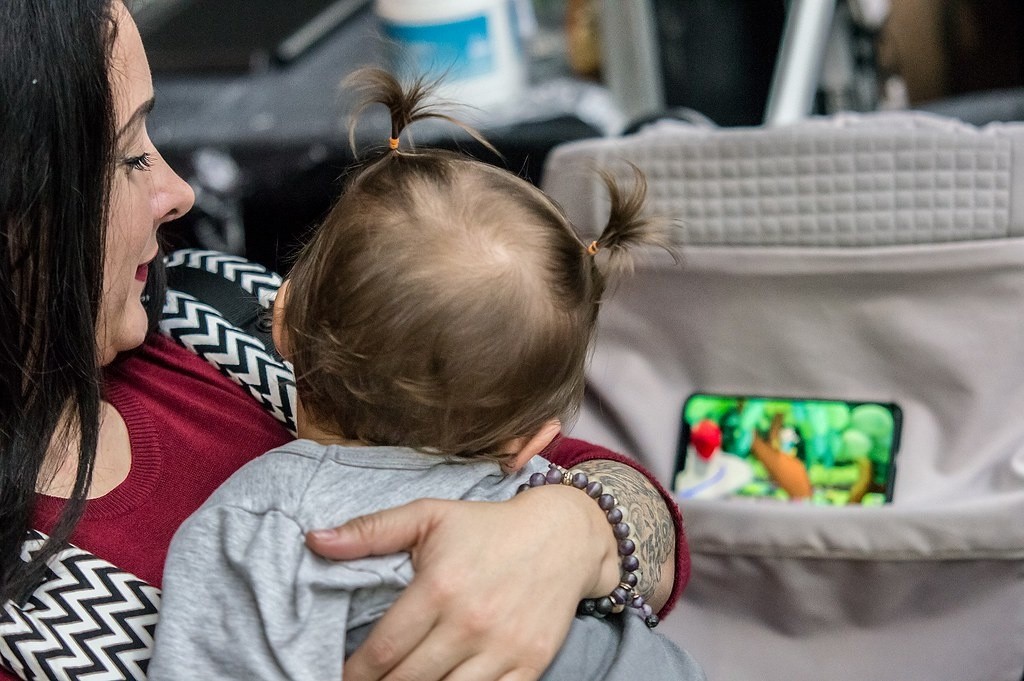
[541,107,1024,679]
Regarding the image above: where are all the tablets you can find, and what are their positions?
[670,389,903,505]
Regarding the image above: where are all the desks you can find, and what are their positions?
[149,1,567,146]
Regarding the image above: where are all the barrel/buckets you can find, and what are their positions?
[376,0,526,122]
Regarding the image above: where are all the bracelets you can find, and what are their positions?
[512,469,660,631]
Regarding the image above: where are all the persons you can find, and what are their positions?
[0,0,690,681]
[148,59,695,681]
